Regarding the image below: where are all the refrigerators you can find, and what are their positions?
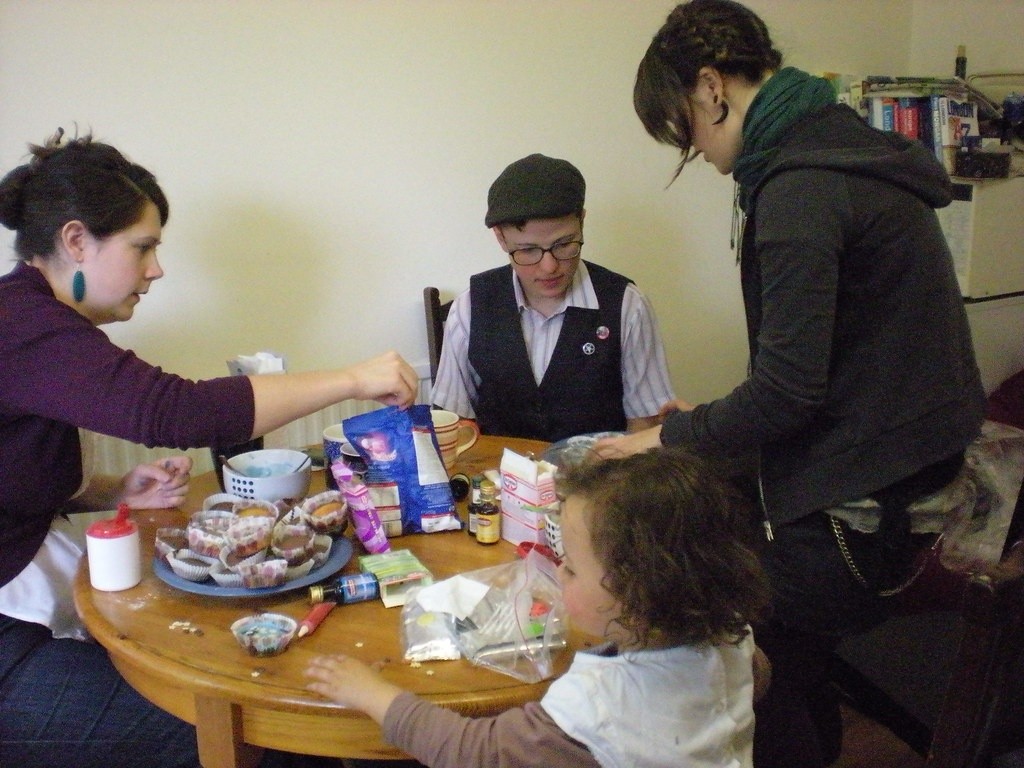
[934,175,1024,398]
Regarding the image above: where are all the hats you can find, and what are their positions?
[485,153,586,228]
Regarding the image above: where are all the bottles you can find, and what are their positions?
[84,503,143,592]
[468,476,484,536]
[449,471,470,502]
[953,45,967,81]
[476,480,500,545]
[308,573,380,606]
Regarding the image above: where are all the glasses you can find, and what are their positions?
[500,213,584,266]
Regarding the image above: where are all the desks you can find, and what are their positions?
[71,429,614,767]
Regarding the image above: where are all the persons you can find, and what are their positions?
[586,0,987,768]
[428,152,678,437]
[301,445,772,768]
[0,126,420,768]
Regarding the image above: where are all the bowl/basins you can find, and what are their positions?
[222,449,311,505]
[230,613,298,657]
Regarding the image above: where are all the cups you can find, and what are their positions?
[430,410,480,470]
[323,423,349,492]
[340,443,369,484]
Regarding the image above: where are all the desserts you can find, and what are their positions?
[231,613,298,658]
[155,490,349,589]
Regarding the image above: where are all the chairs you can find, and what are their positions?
[796,539,1024,768]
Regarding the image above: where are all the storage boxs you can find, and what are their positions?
[500,447,562,547]
